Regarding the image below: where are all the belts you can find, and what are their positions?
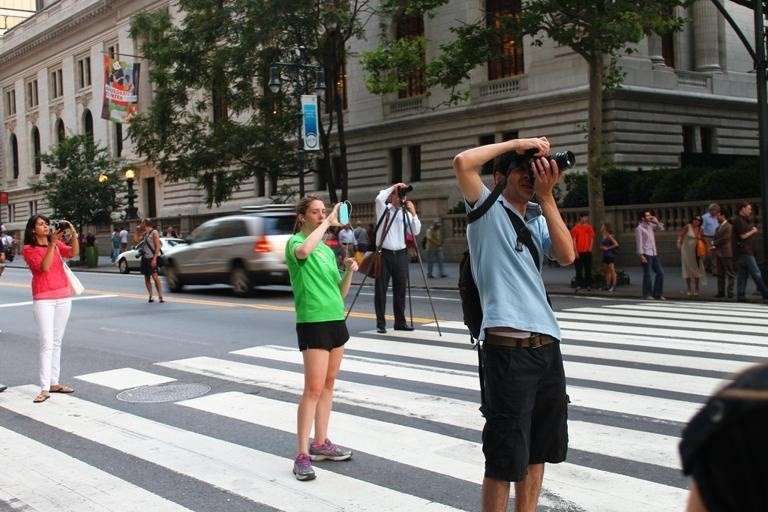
[483,331,558,350]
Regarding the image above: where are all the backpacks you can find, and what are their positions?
[421,236,427,250]
[458,247,483,344]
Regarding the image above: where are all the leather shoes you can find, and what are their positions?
[376,326,387,333]
[643,294,667,301]
[687,291,700,296]
[394,323,415,331]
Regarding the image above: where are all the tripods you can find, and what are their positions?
[345,201,441,337]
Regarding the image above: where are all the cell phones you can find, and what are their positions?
[339,204,349,224]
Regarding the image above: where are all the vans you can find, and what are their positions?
[156,205,343,295]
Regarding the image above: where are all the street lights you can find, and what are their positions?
[675,0,766,295]
[123,168,140,237]
[265,44,325,205]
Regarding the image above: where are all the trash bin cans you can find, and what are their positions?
[85,246,98,268]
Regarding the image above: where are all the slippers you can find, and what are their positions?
[33,393,50,402]
[48,386,74,393]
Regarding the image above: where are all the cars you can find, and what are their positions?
[113,236,187,280]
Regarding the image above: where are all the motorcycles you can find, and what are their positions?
[0,239,19,263]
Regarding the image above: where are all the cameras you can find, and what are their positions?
[55,221,70,234]
[398,185,413,197]
[135,249,146,259]
[525,148,575,184]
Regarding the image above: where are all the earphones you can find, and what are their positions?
[302,218,305,222]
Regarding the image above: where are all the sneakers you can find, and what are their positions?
[158,298,165,303]
[427,274,448,278]
[309,438,354,462]
[292,453,317,481]
[148,297,154,302]
[603,285,617,292]
[575,285,592,291]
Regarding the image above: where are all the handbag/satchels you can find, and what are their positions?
[696,238,708,256]
[61,261,85,297]
[357,250,382,279]
[156,253,170,268]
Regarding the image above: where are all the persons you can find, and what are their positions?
[407,232,419,262]
[676,203,767,304]
[569,213,620,292]
[284,193,362,483]
[108,69,123,107]
[111,228,128,264]
[450,135,579,512]
[674,358,768,511]
[424,219,448,278]
[19,212,83,404]
[371,182,423,336]
[124,74,134,93]
[321,222,369,271]
[1,225,15,277]
[132,218,179,303]
[87,232,98,246]
[635,211,665,300]
